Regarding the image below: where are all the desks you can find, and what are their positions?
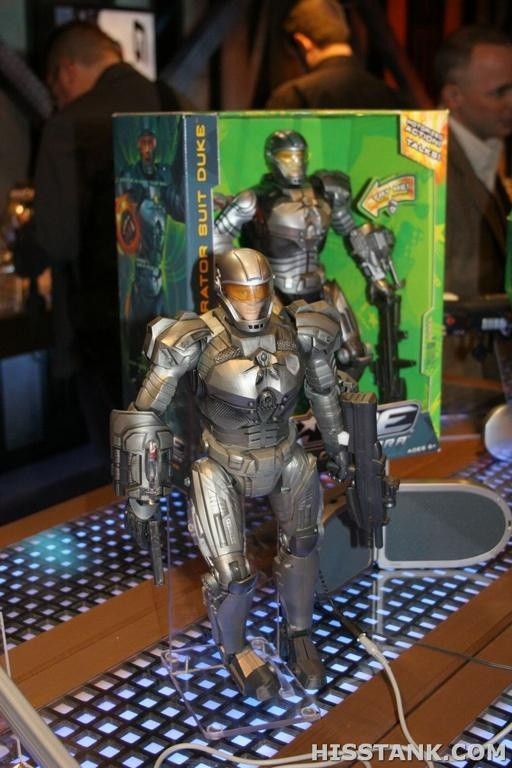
[443,360,512,415]
[0,415,512,768]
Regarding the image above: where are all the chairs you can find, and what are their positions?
[0,312,117,523]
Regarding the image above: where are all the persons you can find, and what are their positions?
[13,16,169,477]
[109,243,400,701]
[113,127,187,410]
[425,20,512,383]
[212,125,418,406]
[1,40,53,221]
[262,0,407,111]
